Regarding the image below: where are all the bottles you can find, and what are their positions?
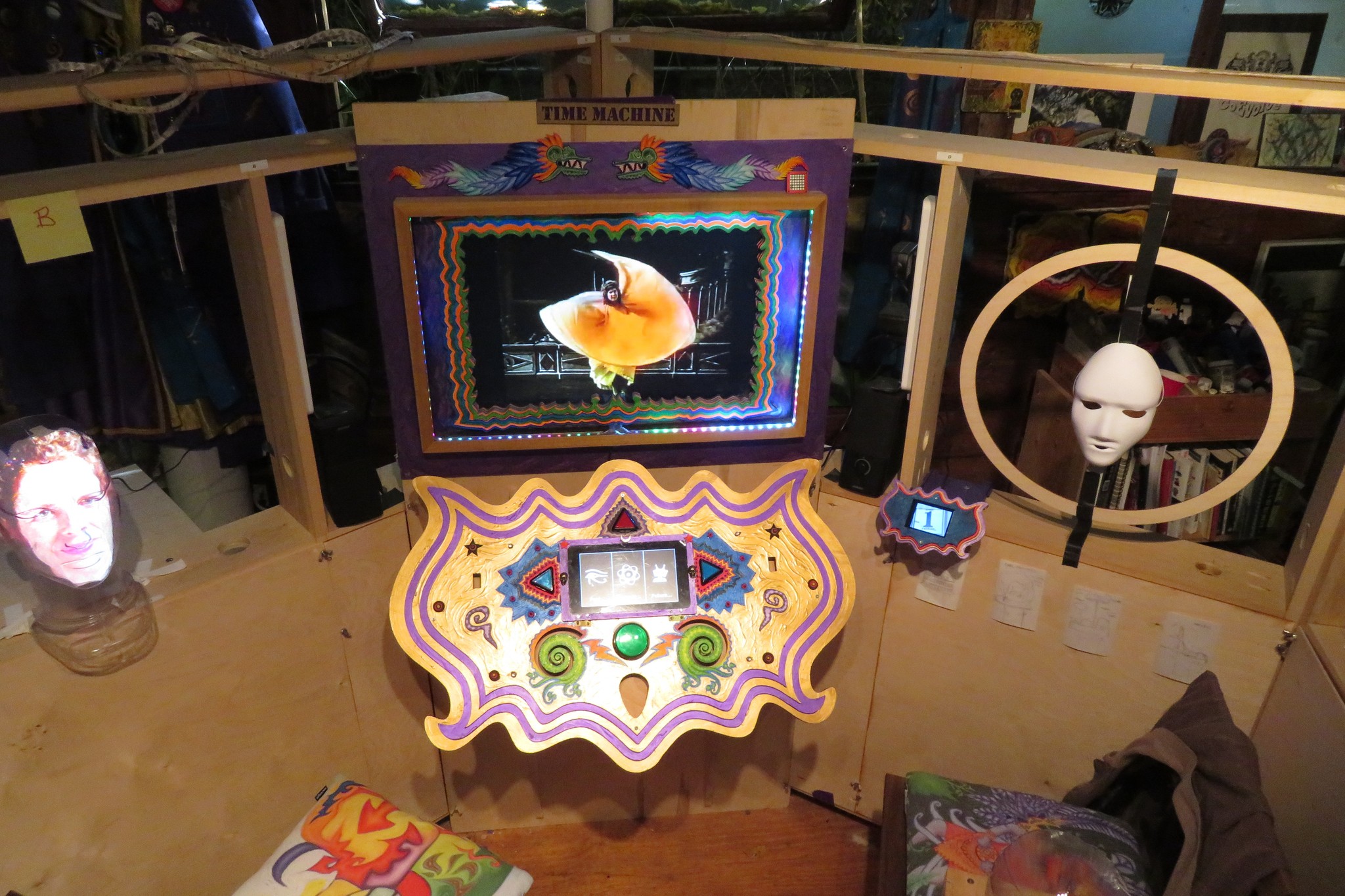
[1208,360,1235,394]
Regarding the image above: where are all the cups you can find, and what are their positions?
[1159,368,1190,397]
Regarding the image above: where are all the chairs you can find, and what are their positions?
[877,670,1298,896]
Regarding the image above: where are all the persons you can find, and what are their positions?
[0,410,159,676]
[601,281,630,314]
